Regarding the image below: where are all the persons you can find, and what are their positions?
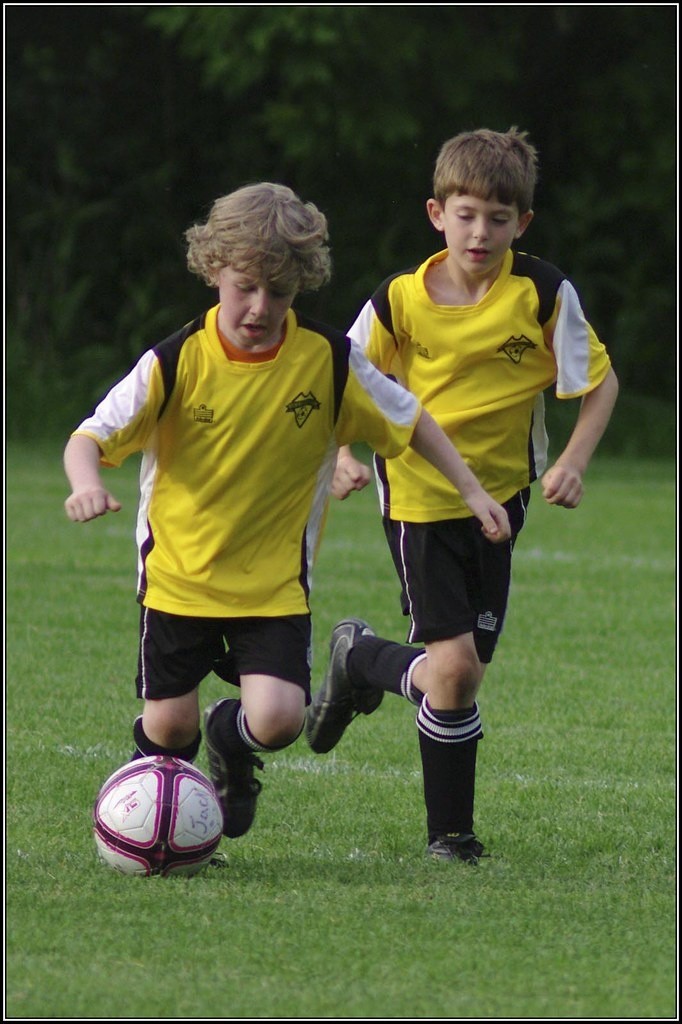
[65,185,512,867]
[305,128,620,860]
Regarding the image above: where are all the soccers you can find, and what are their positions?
[95,754,224,881]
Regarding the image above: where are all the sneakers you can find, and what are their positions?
[303,618,383,754]
[203,697,266,838]
[206,852,229,870]
[428,831,511,876]
